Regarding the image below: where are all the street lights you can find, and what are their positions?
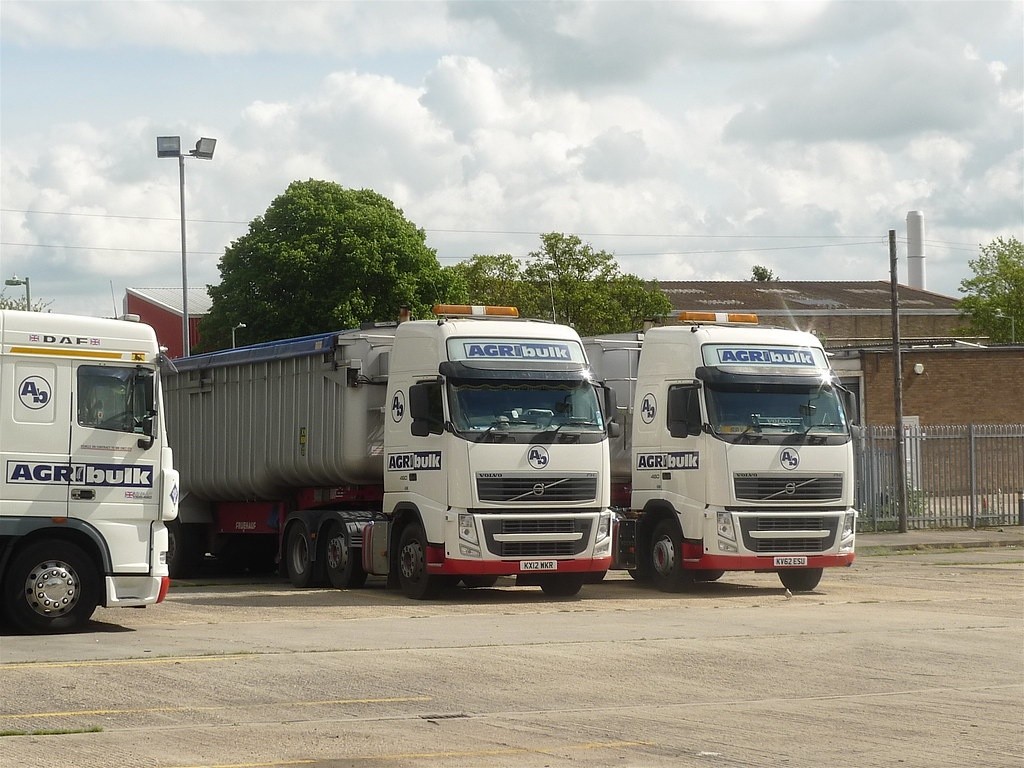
[4,274,31,310]
[155,132,217,364]
[229,322,248,347]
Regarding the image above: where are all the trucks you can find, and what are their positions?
[2,309,183,635]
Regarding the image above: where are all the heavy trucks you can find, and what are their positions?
[161,303,632,599]
[577,311,863,593]
